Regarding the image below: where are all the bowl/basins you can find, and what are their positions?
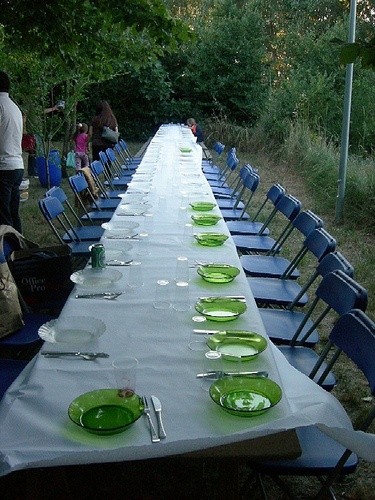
[175,123,282,418]
[67,388,144,436]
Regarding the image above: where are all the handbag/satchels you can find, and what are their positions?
[101,126,120,144]
[22,134,36,150]
[8,243,74,305]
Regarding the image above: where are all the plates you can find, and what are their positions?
[37,123,170,346]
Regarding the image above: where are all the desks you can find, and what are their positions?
[0,123,375,500]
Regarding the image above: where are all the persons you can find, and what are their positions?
[75,101,119,180]
[0,71,25,250]
[187,118,203,142]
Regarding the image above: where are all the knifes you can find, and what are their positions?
[41,351,109,357]
[149,395,166,439]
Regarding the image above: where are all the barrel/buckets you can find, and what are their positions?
[19,178,30,201]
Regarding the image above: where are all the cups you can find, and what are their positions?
[138,233,150,256]
[128,261,144,287]
[200,351,228,393]
[186,316,210,351]
[152,279,171,309]
[173,257,189,280]
[112,356,139,397]
[179,206,187,222]
[183,224,194,245]
[172,282,193,311]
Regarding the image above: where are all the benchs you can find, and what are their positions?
[135,137,153,158]
[204,150,212,159]
[200,142,208,150]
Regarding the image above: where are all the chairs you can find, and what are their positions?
[0,139,143,400]
[202,142,375,500]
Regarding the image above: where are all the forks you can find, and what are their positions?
[139,394,160,443]
